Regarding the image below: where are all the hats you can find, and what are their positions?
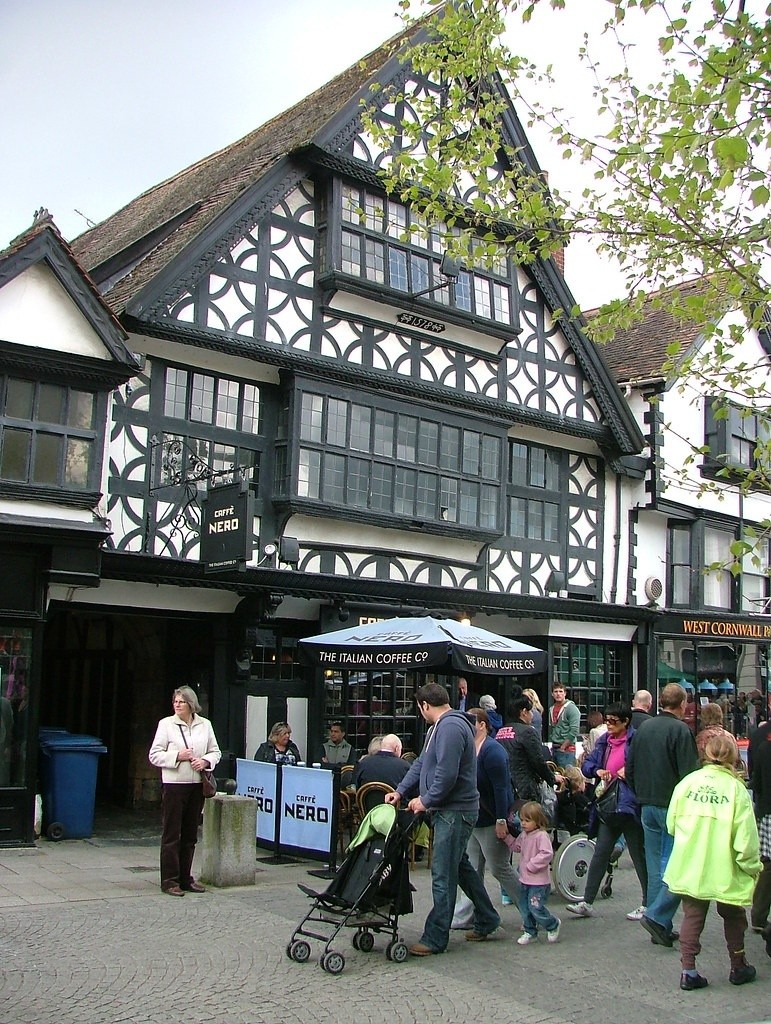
[479,694,497,710]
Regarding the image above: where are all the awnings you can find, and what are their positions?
[557,643,698,686]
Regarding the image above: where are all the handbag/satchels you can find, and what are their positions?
[200,769,217,798]
[594,776,621,813]
[506,798,532,827]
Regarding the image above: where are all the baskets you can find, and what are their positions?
[735,760,749,782]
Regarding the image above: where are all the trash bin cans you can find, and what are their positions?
[39,730,109,842]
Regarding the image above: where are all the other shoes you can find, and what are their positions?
[517,931,538,945]
[184,881,206,893]
[761,923,771,957]
[450,921,475,930]
[520,920,539,930]
[164,885,185,897]
[729,962,756,985]
[409,942,433,956]
[651,929,680,945]
[464,926,498,941]
[751,919,769,930]
[680,973,708,991]
[639,915,674,947]
[406,849,425,862]
[548,918,561,942]
[501,896,514,905]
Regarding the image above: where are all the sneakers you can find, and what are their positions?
[626,905,648,920]
[567,901,593,917]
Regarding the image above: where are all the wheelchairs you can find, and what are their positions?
[544,760,620,903]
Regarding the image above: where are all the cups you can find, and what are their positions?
[297,761,306,767]
[312,762,321,769]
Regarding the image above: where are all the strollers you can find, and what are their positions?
[287,795,435,975]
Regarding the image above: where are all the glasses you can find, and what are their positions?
[276,722,288,730]
[603,715,621,725]
[171,699,188,706]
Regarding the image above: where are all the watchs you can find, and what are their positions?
[497,819,506,824]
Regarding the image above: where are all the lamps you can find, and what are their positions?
[409,250,460,299]
[544,570,564,598]
[278,536,300,571]
[673,677,734,690]
[257,543,278,566]
[336,605,350,623]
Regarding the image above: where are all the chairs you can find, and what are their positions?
[332,752,432,871]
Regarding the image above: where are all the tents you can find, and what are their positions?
[296,616,550,711]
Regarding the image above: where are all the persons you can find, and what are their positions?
[385,683,500,954]
[254,721,302,766]
[631,689,653,729]
[565,702,647,921]
[746,721,771,956]
[318,721,357,788]
[696,702,741,762]
[148,686,221,896]
[663,737,766,991]
[712,689,767,738]
[352,733,424,862]
[553,764,599,840]
[623,683,696,950]
[581,710,608,754]
[449,675,581,944]
